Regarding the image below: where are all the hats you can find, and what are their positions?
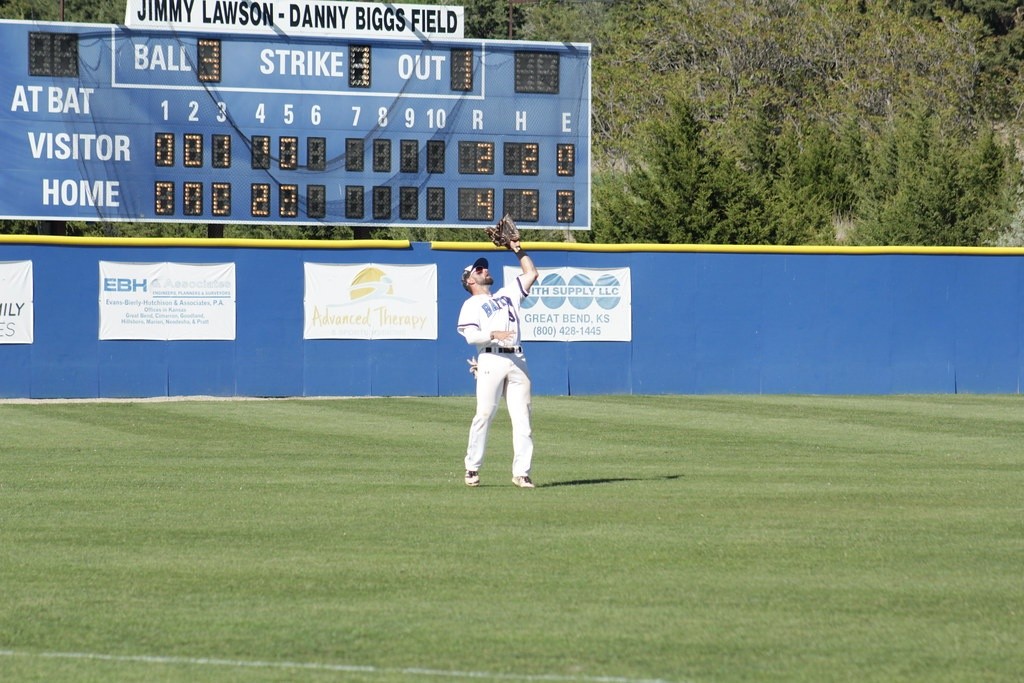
[461,258,489,286]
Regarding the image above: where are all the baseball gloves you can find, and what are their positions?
[485,213,521,249]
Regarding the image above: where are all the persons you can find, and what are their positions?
[456,211,539,487]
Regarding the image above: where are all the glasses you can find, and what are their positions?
[467,266,484,285]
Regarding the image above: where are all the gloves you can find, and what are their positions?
[466,356,478,379]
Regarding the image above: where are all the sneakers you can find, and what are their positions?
[512,476,535,487]
[465,470,479,486]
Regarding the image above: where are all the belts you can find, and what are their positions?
[486,347,522,353]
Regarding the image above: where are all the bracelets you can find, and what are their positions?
[513,246,528,260]
